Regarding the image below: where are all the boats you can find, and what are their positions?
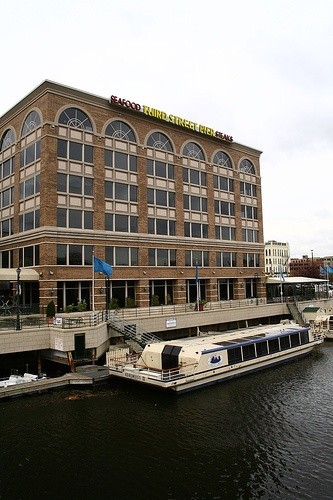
[111,317,324,390]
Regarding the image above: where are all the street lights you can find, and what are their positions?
[16,265,21,331]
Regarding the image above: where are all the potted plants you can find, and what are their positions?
[194,300,207,311]
[46,300,55,323]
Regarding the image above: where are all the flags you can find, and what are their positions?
[321,266,327,274]
[329,266,333,274]
[94,256,113,277]
